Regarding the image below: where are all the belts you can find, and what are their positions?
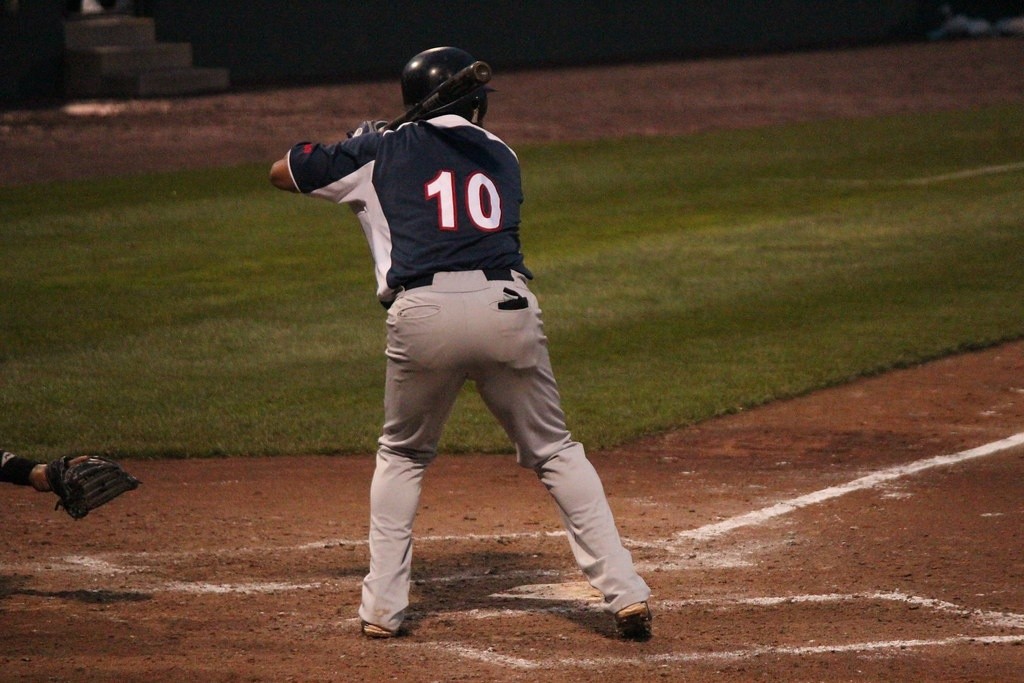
[394,268,515,294]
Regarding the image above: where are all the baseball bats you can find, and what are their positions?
[377,60,491,132]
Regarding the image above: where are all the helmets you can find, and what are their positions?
[400,46,488,123]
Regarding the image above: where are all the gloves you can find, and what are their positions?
[346,119,388,139]
[498,287,529,310]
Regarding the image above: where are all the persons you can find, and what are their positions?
[0,450,138,517]
[270,45,653,640]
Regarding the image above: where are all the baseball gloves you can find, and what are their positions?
[45,455,138,519]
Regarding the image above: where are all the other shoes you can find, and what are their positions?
[360,619,396,637]
[614,601,653,642]
